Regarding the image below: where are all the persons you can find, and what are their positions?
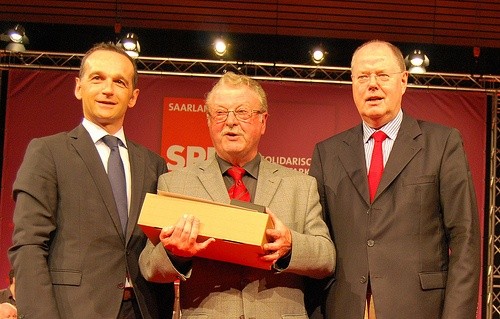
[137,72,335,319]
[0,302,18,319]
[5,40,172,318]
[0,266,17,308]
[308,40,481,318]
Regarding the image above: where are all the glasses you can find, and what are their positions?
[351,71,403,83]
[207,106,263,123]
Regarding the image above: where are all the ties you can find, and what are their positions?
[368,131,388,204]
[102,135,128,240]
[226,166,251,204]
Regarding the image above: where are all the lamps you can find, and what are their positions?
[213,37,228,56]
[404,46,429,67]
[115,31,141,57]
[0,24,29,45]
[308,43,330,65]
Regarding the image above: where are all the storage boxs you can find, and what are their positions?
[137,189,275,271]
